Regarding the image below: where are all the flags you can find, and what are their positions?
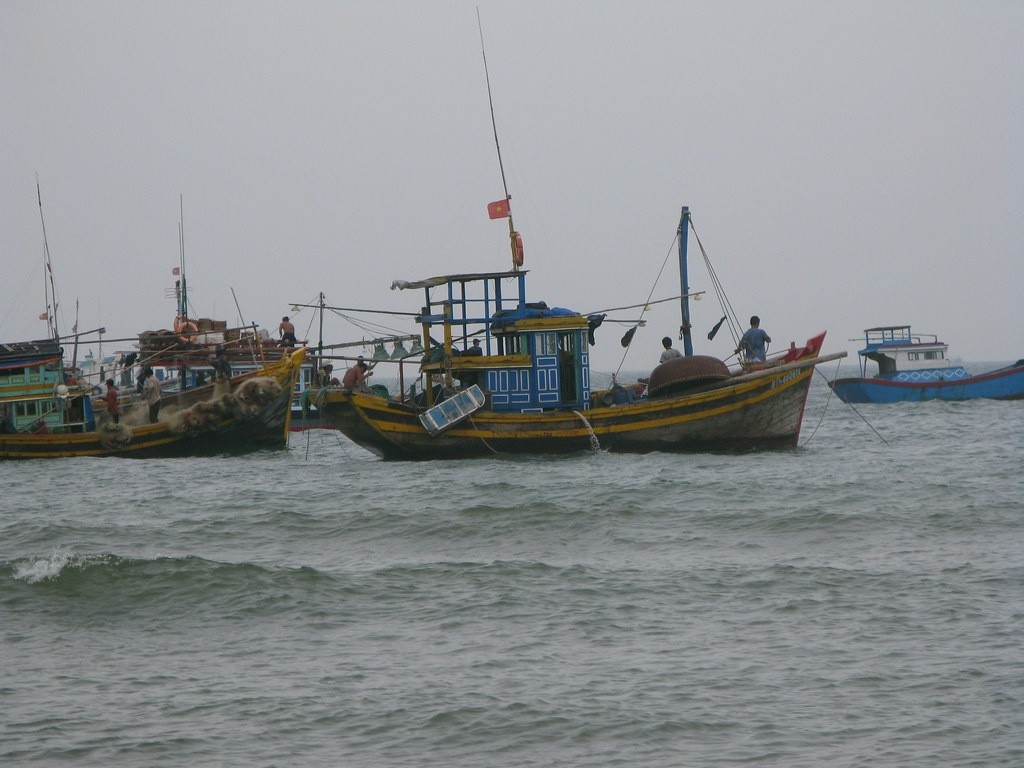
[173,267,179,275]
[39,313,47,320]
[487,199,509,219]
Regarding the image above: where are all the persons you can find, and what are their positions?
[279,316,295,347]
[739,316,771,361]
[143,369,161,424]
[469,339,482,356]
[343,356,379,389]
[98,379,119,424]
[659,336,684,363]
[208,347,231,379]
[323,365,341,386]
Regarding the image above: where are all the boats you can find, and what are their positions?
[0,176,345,460]
[828,326,1024,404]
[301,6,837,468]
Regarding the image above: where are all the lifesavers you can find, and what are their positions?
[91,386,103,395]
[173,315,191,332]
[177,322,199,342]
[601,391,615,406]
